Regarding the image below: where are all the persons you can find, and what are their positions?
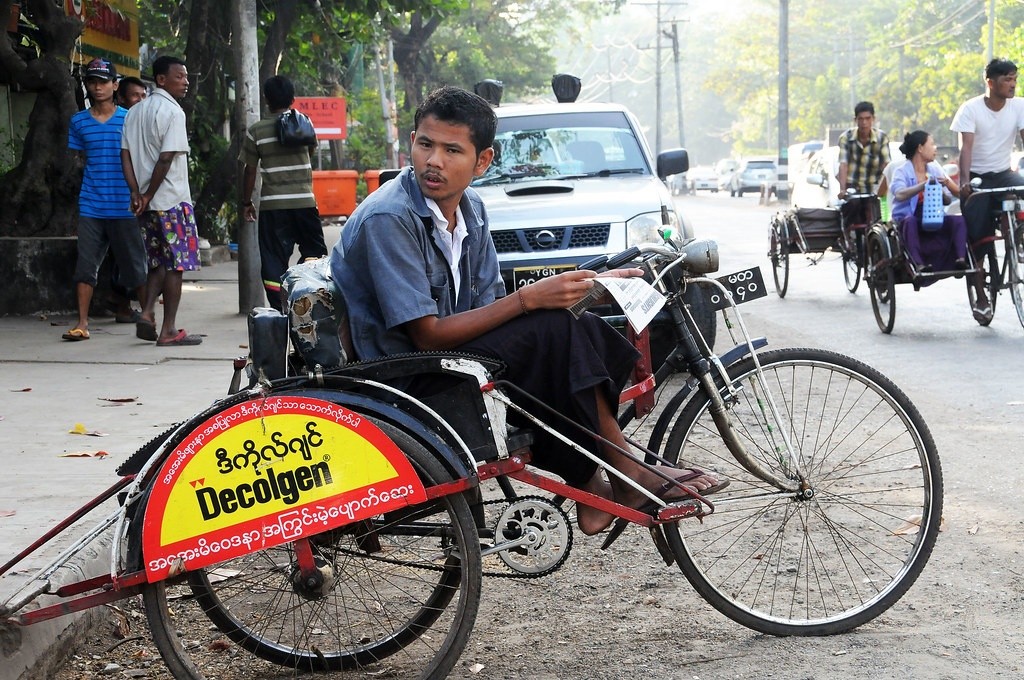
[328,85,730,537]
[236,76,327,314]
[837,102,891,280]
[948,59,1023,325]
[876,158,952,221]
[891,130,969,282]
[120,56,202,346]
[61,57,149,341]
[88,77,147,324]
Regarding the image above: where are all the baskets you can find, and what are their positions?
[921,175,945,232]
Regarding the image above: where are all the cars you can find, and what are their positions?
[668,130,845,210]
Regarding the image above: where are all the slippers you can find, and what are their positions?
[973,300,992,325]
[136,319,158,340]
[600,469,730,551]
[62,329,89,341]
[156,329,202,346]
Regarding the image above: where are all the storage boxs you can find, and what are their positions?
[363,169,382,195]
[308,166,359,217]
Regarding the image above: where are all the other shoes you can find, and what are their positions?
[953,260,967,278]
[917,265,934,280]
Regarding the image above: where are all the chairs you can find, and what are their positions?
[280,251,529,467]
[872,192,978,286]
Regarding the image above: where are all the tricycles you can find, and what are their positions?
[3,253,946,678]
[858,173,1024,336]
[761,182,906,336]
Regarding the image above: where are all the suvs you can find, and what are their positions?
[374,100,720,380]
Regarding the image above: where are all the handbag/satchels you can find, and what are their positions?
[275,107,317,146]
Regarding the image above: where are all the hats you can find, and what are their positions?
[84,57,116,81]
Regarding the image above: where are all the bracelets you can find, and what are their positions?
[519,287,528,314]
